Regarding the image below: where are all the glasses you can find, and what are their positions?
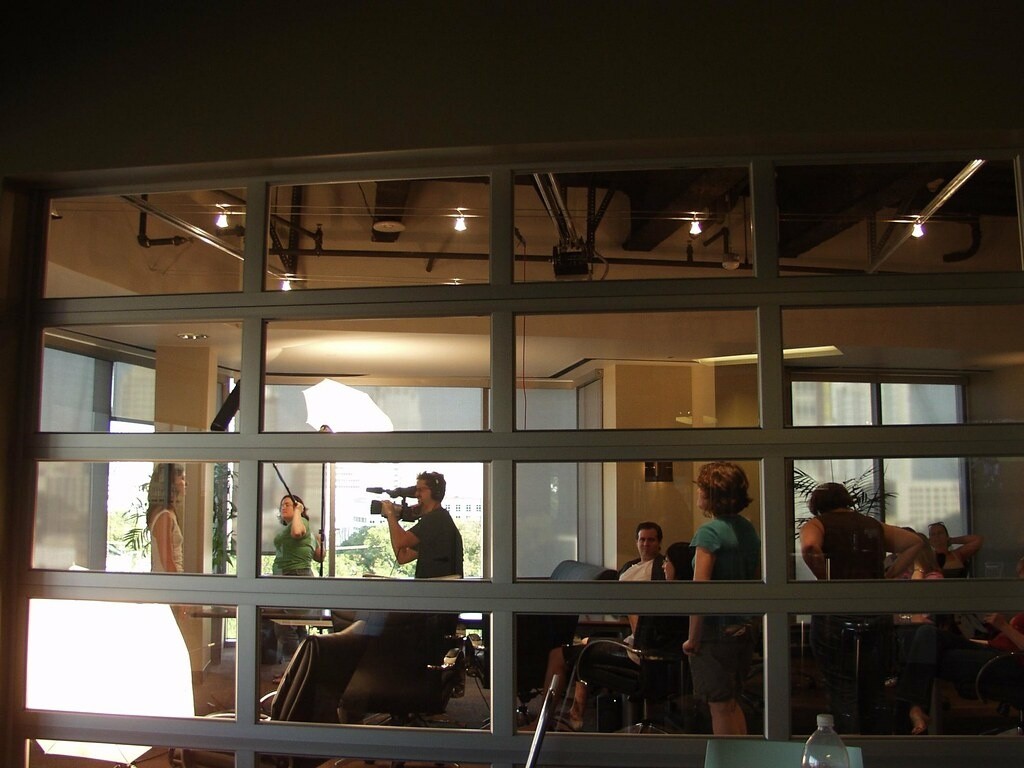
[928,522,945,531]
[416,487,432,493]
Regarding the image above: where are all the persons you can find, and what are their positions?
[383,461,1024,735]
[144,464,187,573]
[271,496,327,683]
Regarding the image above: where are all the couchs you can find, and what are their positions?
[484,560,616,689]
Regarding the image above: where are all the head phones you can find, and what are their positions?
[430,475,444,500]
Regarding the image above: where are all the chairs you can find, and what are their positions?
[975,651,1023,736]
[166,622,366,768]
[574,615,687,732]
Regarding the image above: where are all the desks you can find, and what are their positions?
[273,619,332,629]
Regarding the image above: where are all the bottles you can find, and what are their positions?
[800,714,850,768]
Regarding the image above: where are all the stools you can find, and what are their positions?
[833,615,881,709]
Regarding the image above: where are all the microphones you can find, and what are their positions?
[211,381,239,431]
[366,487,388,494]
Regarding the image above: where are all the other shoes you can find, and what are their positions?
[960,688,979,700]
[272,672,282,683]
[908,706,932,736]
[553,711,581,732]
